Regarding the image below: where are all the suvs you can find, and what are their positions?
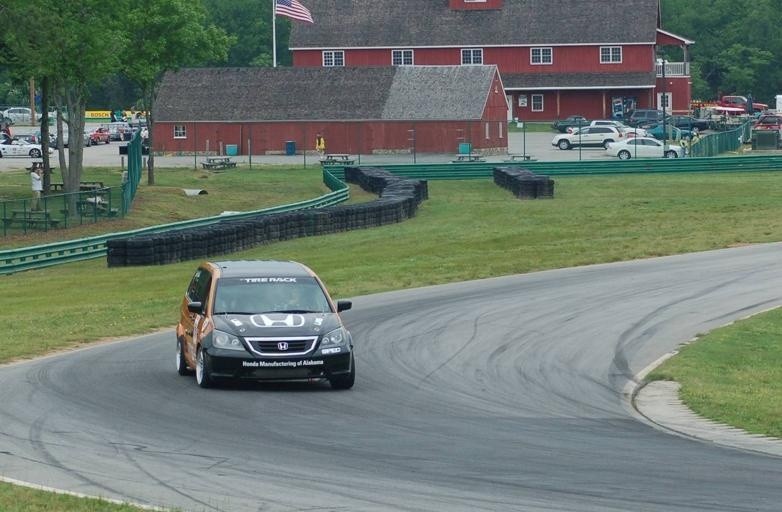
[721,95,768,116]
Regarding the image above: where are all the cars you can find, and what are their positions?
[176,259,355,389]
[0,122,133,158]
[551,108,710,150]
[3,107,40,126]
[606,137,684,158]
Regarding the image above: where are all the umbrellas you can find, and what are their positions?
[744,89,754,116]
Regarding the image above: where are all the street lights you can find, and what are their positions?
[658,49,665,145]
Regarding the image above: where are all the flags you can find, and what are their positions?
[275,0,314,24]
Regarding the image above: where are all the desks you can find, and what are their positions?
[326,154,350,166]
[507,154,533,160]
[456,153,482,160]
[206,155,232,170]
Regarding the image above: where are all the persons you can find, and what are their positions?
[118,125,125,141]
[315,132,326,161]
[31,166,45,211]
[142,128,149,138]
[110,109,118,122]
[130,105,136,119]
[120,109,128,122]
[283,284,308,306]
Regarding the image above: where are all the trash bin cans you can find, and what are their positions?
[459,143,472,154]
[226,144,237,156]
[286,141,295,156]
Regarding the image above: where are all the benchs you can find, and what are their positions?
[318,159,355,166]
[200,161,237,170]
[0,181,120,230]
[451,160,486,163]
